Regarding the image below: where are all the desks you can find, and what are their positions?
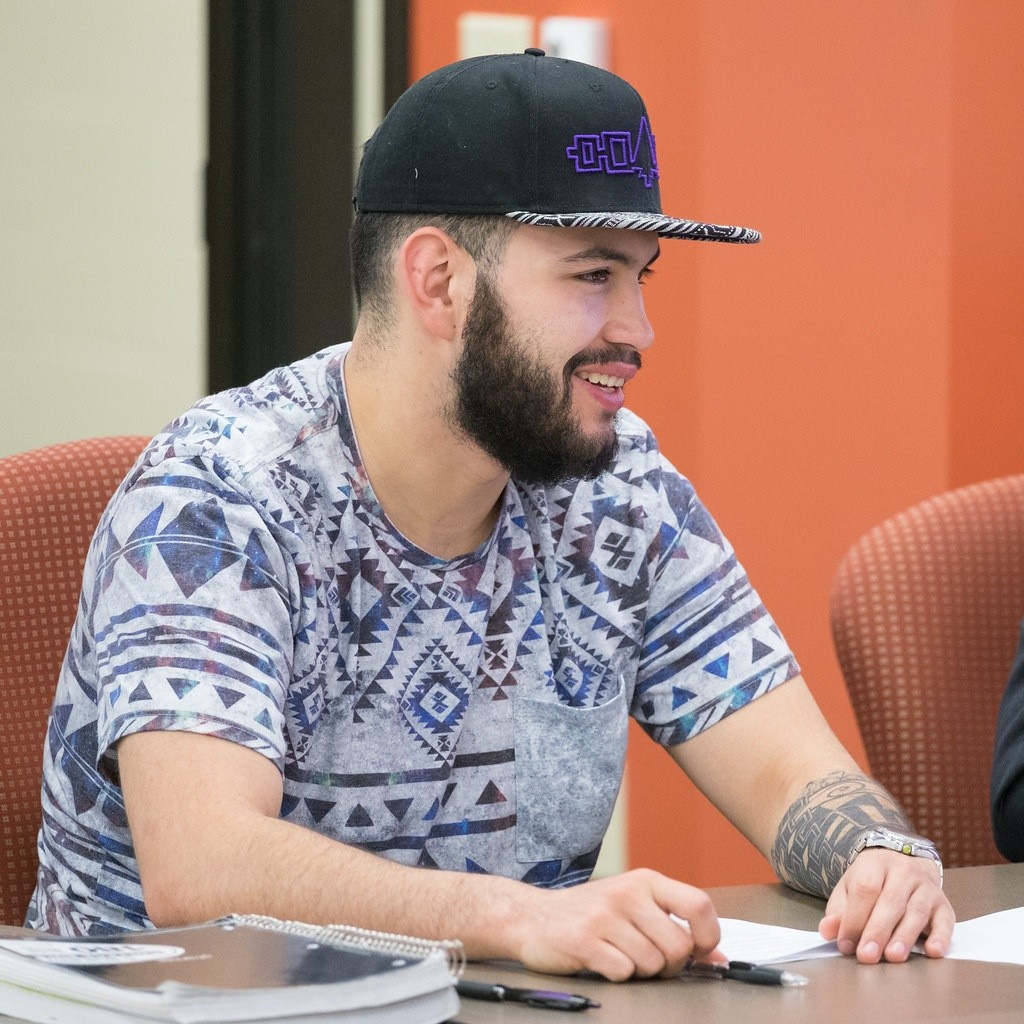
[447,863,1024,1024]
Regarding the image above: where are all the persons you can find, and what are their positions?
[22,48,955,983]
[990,596,1024,862]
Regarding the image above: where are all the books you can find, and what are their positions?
[0,913,463,1024]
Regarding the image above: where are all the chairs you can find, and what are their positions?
[0,434,153,933]
[834,474,1024,870]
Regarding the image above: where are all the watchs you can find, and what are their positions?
[838,826,943,894]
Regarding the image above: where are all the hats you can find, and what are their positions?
[350,47,762,244]
